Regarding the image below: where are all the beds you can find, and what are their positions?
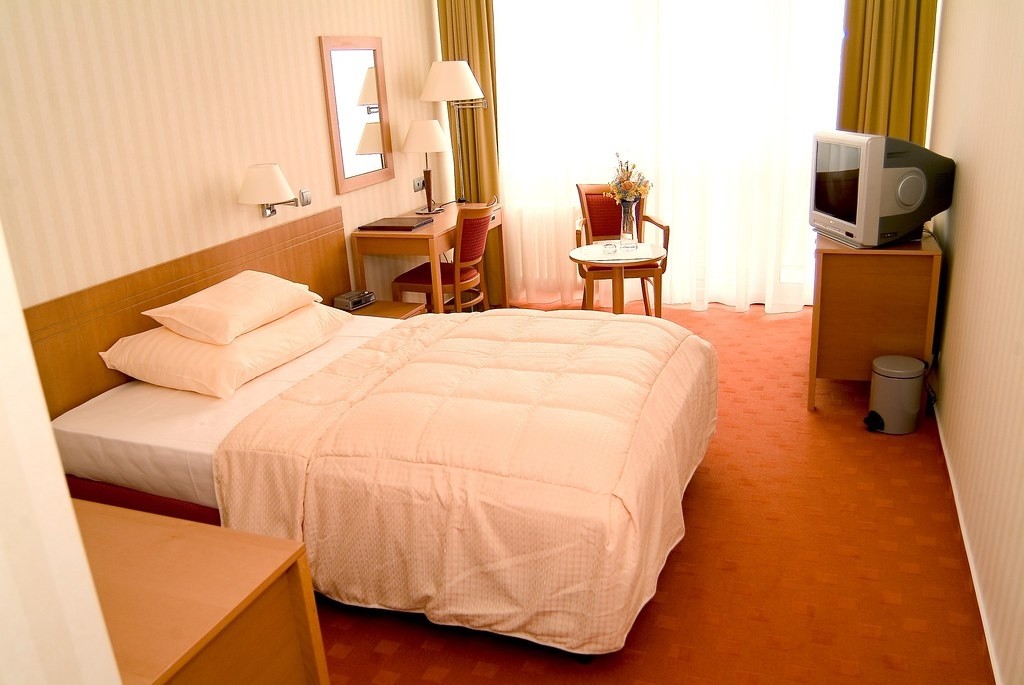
[52,314,719,662]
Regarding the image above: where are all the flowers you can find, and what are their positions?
[603,152,653,231]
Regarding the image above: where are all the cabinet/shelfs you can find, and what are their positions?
[807,223,943,411]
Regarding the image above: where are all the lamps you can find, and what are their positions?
[356,67,379,115]
[402,119,450,213]
[356,122,385,169]
[420,60,488,204]
[236,163,298,218]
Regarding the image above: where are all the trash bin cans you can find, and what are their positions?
[863,355,928,435]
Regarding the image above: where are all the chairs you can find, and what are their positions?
[392,195,498,314]
[576,182,670,318]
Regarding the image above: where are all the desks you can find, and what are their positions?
[350,202,502,315]
[569,239,667,315]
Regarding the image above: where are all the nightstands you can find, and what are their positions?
[350,301,427,321]
[71,497,331,685]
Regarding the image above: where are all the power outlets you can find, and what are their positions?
[413,176,426,192]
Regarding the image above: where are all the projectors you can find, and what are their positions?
[334,290,377,311]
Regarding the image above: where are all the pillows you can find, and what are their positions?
[141,269,323,346]
[98,301,355,400]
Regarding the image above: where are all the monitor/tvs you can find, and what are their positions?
[809,129,956,246]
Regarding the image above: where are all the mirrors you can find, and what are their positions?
[318,35,395,195]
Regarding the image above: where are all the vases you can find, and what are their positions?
[620,200,640,249]
